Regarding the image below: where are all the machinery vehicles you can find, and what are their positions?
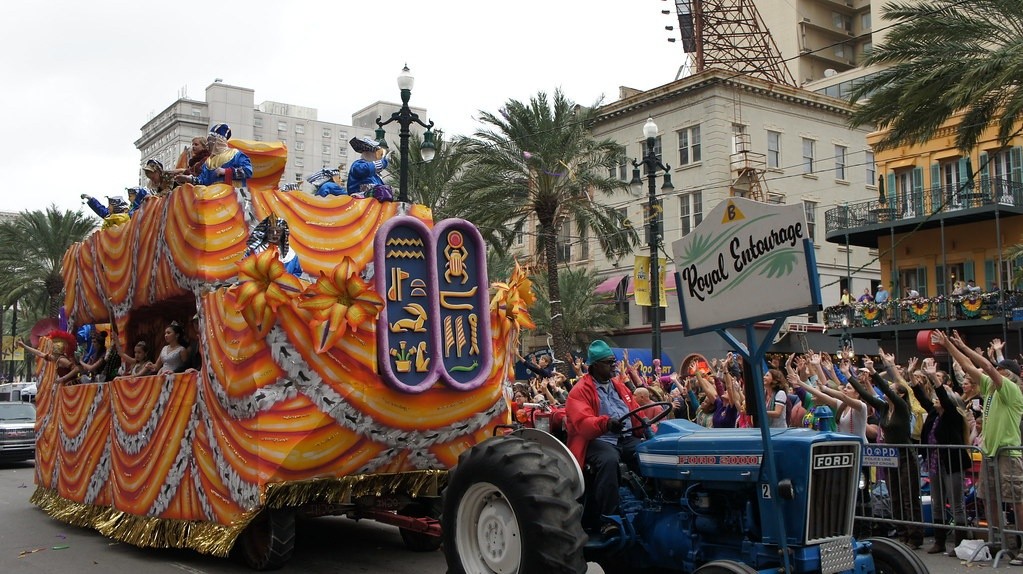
[434,400,931,574]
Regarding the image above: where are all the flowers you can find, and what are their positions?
[491,256,538,345]
[822,287,1019,326]
[233,242,386,355]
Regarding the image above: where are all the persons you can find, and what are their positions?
[858,284,889,327]
[836,288,856,328]
[82,123,395,231]
[967,279,975,288]
[905,286,920,324]
[510,329,1022,557]
[564,339,658,541]
[17,315,202,386]
[990,283,1000,303]
[234,211,302,285]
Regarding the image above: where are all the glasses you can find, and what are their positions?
[513,395,524,400]
[597,360,616,365]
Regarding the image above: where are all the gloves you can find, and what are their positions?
[607,417,626,435]
[384,151,396,160]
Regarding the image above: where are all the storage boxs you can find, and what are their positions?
[921,496,934,538]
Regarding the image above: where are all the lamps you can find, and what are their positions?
[662,0,682,42]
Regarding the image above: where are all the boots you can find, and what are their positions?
[926,519,947,553]
[949,523,967,556]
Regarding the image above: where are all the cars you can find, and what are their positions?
[0,399,37,465]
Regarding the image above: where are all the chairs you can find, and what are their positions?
[886,203,908,219]
[849,208,868,227]
[550,410,628,474]
[942,487,980,538]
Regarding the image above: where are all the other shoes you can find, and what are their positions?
[600,523,619,537]
[906,542,919,550]
[1009,550,1023,566]
[986,547,1004,559]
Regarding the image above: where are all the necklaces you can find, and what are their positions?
[600,381,610,384]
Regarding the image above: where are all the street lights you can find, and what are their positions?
[627,112,675,378]
[373,62,436,203]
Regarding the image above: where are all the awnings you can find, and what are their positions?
[625,272,681,301]
[984,145,1014,152]
[590,274,628,304]
[936,154,963,161]
[891,164,917,170]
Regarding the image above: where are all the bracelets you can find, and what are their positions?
[822,385,826,391]
[885,365,891,368]
[723,372,729,374]
[891,363,895,365]
[91,197,92,200]
[695,372,700,376]
[122,352,124,357]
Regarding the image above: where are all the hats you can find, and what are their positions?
[990,359,1020,377]
[349,136,381,153]
[588,340,614,367]
[278,181,303,193]
[877,285,883,288]
[307,164,344,188]
[245,211,289,258]
[125,187,140,196]
[969,279,975,282]
[105,196,129,214]
[209,123,231,142]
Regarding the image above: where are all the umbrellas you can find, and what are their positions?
[878,172,886,209]
[966,155,976,201]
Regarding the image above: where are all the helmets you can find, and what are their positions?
[48,330,78,358]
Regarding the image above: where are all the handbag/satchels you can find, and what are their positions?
[953,539,992,562]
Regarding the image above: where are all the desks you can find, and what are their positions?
[959,192,989,199]
[869,208,897,213]
